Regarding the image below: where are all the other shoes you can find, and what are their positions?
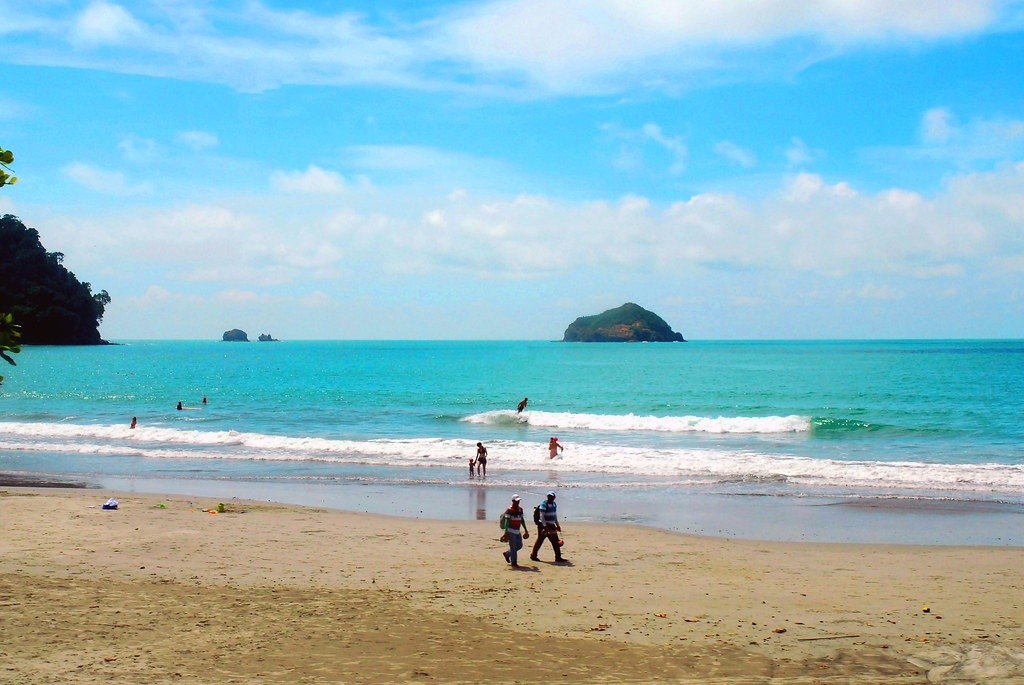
[555,558,567,562]
[503,551,510,563]
[511,563,520,569]
[530,556,540,561]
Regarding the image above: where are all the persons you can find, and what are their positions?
[475,442,487,476]
[469,459,475,477]
[203,398,206,403]
[130,417,136,429]
[517,398,528,413]
[177,402,182,409]
[548,436,563,459]
[530,491,568,562]
[503,495,528,568]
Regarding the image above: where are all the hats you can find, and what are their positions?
[512,494,522,501]
[547,491,556,496]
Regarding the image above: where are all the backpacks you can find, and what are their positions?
[500,508,508,529]
[534,505,541,525]
